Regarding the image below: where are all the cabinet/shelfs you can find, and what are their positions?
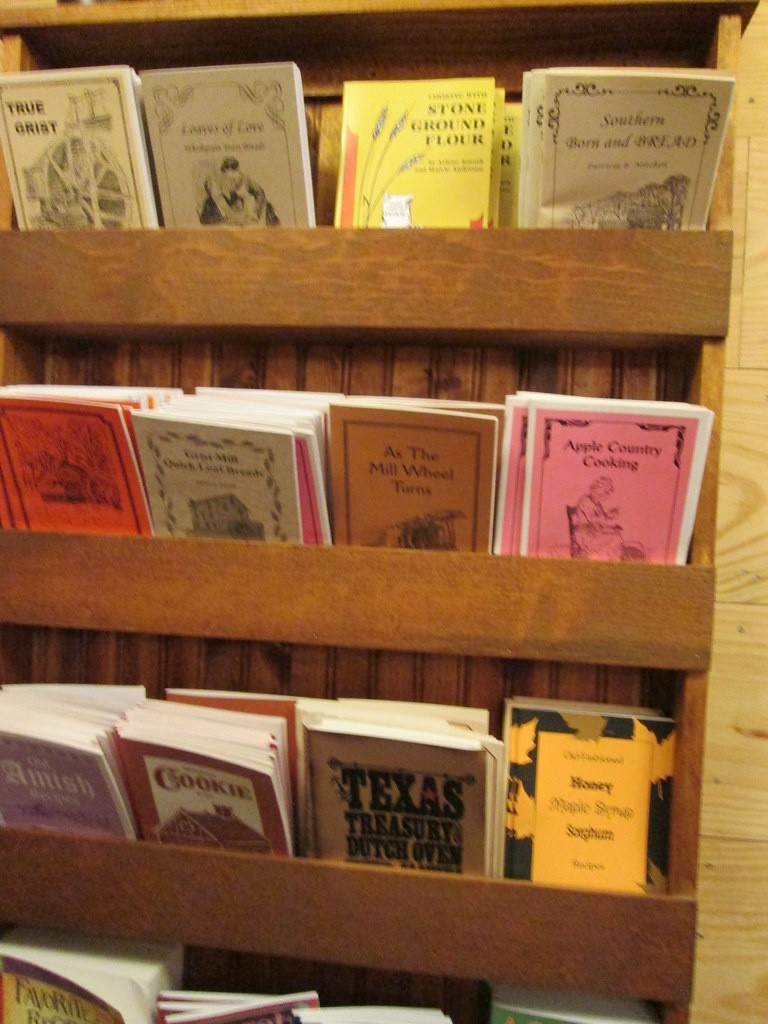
[0,1,758,1024]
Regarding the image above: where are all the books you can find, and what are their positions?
[0,681,677,898]
[0,930,685,1024]
[0,381,714,566]
[0,60,735,233]
